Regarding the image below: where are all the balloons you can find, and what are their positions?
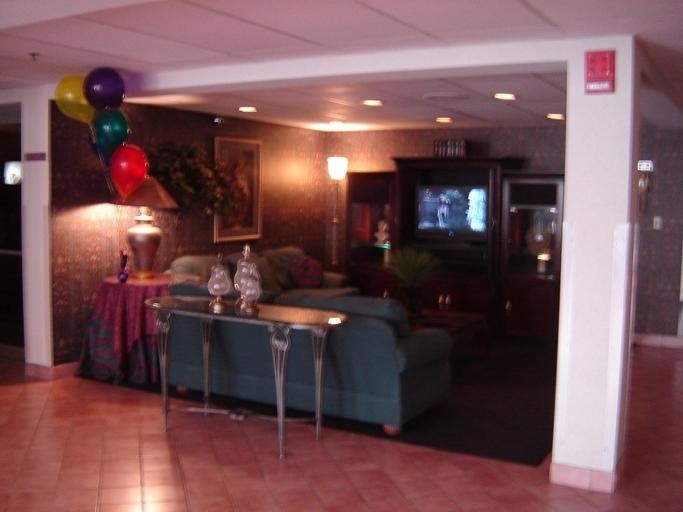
[86,112,130,166]
[109,144,151,206]
[82,67,126,114]
[54,74,96,124]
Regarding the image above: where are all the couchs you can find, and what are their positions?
[164,246,454,437]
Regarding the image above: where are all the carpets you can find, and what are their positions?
[78,339,558,467]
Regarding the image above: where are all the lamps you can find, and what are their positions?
[109,175,177,280]
[326,155,348,272]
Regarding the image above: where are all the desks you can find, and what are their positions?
[100,273,201,385]
[143,295,348,460]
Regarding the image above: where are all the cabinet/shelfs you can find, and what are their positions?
[343,153,564,343]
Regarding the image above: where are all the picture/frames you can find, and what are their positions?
[212,135,263,244]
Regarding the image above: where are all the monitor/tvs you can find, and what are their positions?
[414,184,488,243]
